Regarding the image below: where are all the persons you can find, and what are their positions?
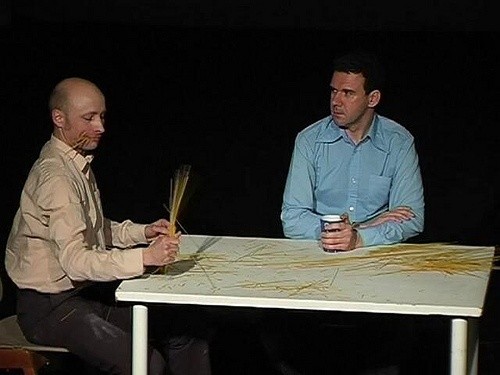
[260,55,425,375]
[3,77,210,375]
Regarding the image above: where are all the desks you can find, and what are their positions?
[116,234,496,375]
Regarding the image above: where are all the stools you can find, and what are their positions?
[0,315,70,375]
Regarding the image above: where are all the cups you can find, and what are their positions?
[320,214,345,253]
